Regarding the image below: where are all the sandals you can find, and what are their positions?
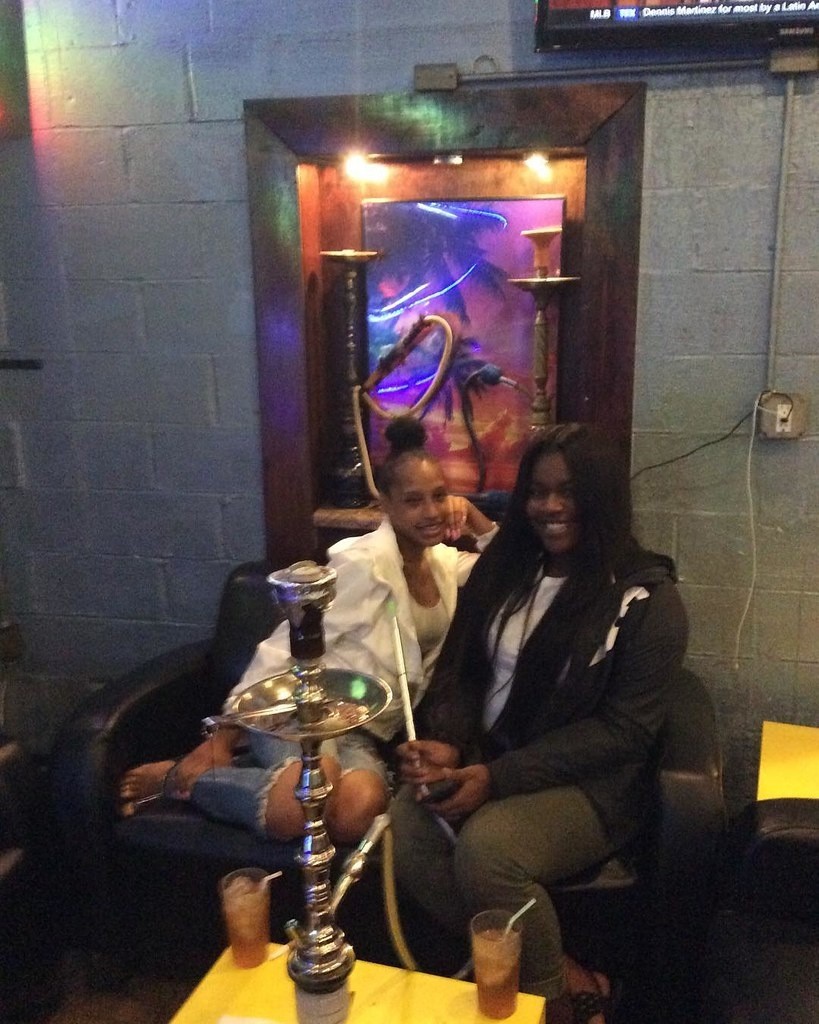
[570,971,623,1023]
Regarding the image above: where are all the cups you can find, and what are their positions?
[220,868,270,969]
[471,910,523,1019]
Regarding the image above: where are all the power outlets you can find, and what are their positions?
[775,404,792,433]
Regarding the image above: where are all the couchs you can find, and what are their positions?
[697,798,819,1024]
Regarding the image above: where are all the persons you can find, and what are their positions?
[121,416,501,845]
[385,424,690,1024]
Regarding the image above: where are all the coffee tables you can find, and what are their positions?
[170,942,548,1024]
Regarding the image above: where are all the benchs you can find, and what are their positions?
[50,564,728,1024]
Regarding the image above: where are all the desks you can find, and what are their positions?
[758,720,819,799]
